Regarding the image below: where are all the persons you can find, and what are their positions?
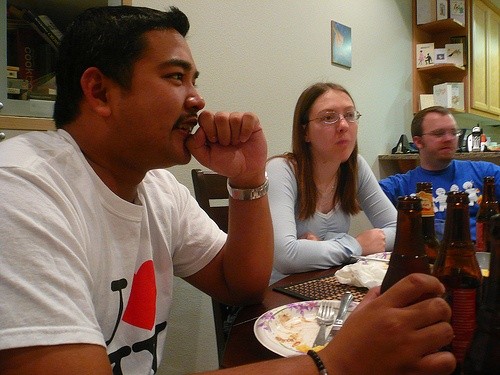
[265,82,398,289]
[0,5,457,375]
[379,106,500,245]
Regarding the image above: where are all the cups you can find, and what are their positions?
[471,132,480,151]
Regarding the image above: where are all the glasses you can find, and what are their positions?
[308,109,361,125]
[422,128,462,138]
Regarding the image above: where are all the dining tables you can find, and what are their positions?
[219,241,490,367]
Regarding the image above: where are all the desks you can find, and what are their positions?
[378,149,500,175]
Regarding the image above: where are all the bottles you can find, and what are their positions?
[432,191,485,375]
[381,196,433,306]
[470,215,500,375]
[416,181,437,266]
[474,176,497,252]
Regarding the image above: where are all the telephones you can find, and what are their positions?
[466,123,486,152]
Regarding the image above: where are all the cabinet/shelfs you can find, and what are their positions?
[412,0,500,128]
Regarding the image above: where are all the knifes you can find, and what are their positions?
[325,293,354,343]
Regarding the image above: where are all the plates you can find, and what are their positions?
[487,146,500,151]
[253,300,359,358]
[359,252,392,270]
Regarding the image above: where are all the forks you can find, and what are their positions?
[311,302,336,350]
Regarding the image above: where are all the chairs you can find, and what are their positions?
[192,170,235,369]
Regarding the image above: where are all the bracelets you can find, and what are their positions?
[227,170,269,200]
[307,350,327,375]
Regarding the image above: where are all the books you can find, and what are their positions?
[7,4,63,100]
